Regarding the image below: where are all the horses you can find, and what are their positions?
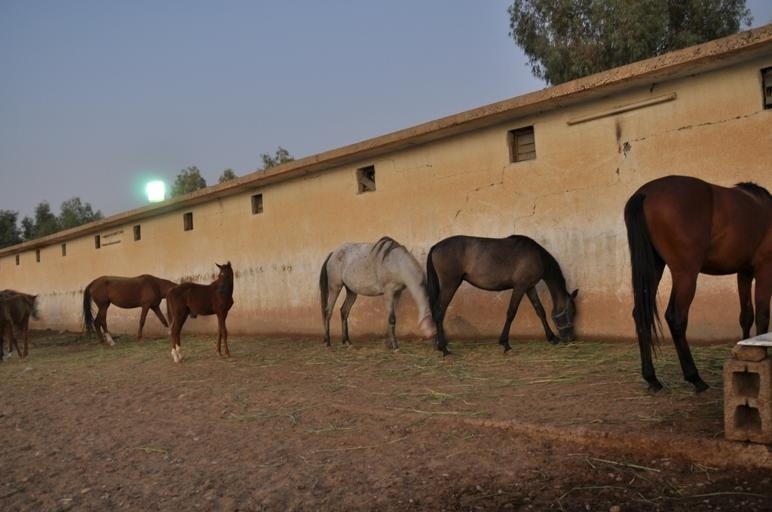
[623,175,772,401]
[80,274,197,347]
[319,236,438,353]
[426,234,579,357]
[166,261,234,363]
[0,289,41,363]
